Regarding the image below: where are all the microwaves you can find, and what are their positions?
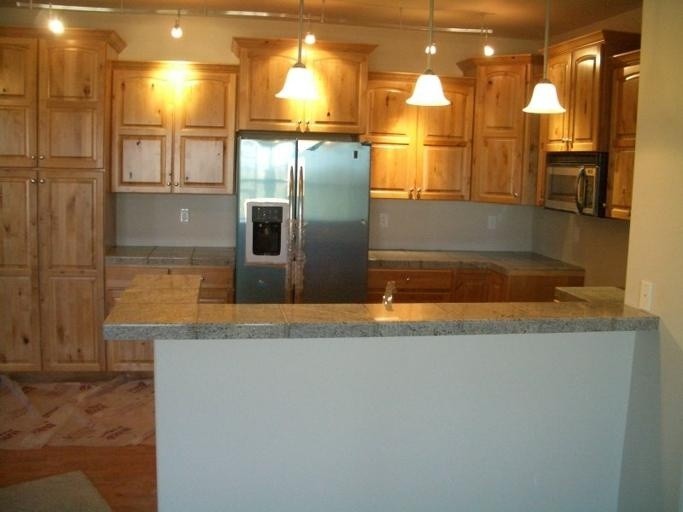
[543,152,606,219]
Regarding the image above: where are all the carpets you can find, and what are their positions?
[1,469,110,511]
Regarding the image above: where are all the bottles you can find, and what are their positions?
[382,281,395,311]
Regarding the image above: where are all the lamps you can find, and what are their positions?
[403,0,453,106]
[275,2,319,101]
[519,1,568,115]
[13,0,498,58]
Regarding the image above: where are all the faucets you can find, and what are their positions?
[380,280,397,304]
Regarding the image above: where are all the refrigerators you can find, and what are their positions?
[235,134,372,305]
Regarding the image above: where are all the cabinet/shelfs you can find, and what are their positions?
[367,269,452,304]
[365,69,474,205]
[108,58,235,195]
[0,169,116,378]
[535,27,639,207]
[604,48,640,221]
[454,268,583,303]
[456,52,538,205]
[103,263,235,376]
[0,26,128,169]
[230,38,378,135]
